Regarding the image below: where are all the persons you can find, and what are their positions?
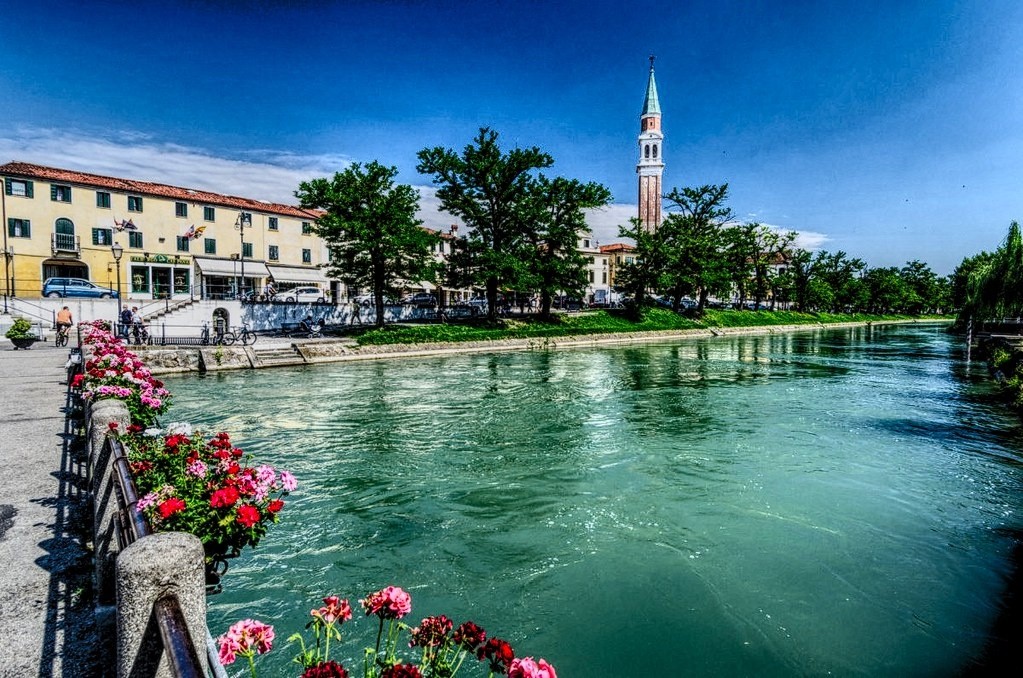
[55,305,73,345]
[130,307,147,346]
[120,303,135,344]
[436,306,448,326]
[350,296,361,328]
[263,281,277,301]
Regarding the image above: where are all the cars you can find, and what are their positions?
[41,277,119,299]
[398,291,436,305]
[466,293,489,306]
[274,287,328,303]
[553,289,796,312]
[354,291,396,306]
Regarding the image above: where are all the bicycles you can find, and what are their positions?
[199,315,257,346]
[54,322,72,346]
[252,290,277,302]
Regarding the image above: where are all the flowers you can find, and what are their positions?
[70,318,559,678]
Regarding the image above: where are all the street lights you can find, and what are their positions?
[111,241,124,324]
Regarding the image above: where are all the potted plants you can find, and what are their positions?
[5,317,37,350]
